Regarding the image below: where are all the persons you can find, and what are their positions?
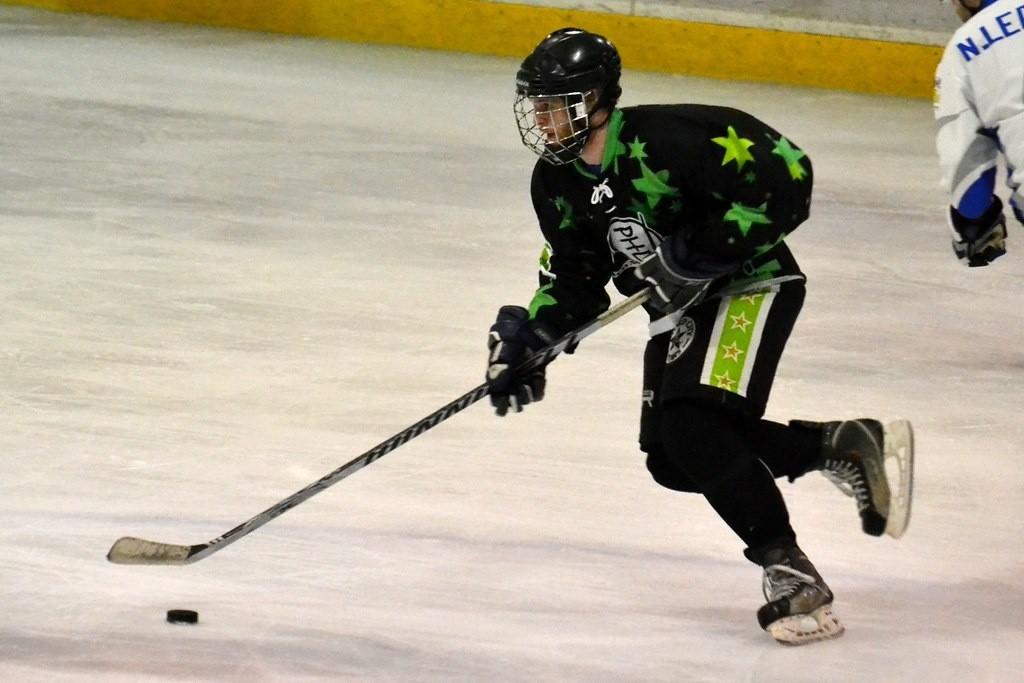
[934,0,1024,267]
[486,28,915,647]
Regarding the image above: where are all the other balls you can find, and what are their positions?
[164,608,200,625]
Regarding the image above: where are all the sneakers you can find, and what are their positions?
[743,524,845,646]
[789,419,914,539]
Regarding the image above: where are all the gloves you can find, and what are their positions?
[613,248,711,322]
[949,196,1006,267]
[486,305,547,417]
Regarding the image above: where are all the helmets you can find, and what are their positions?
[513,27,621,166]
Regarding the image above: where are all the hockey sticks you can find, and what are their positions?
[106,290,658,567]
